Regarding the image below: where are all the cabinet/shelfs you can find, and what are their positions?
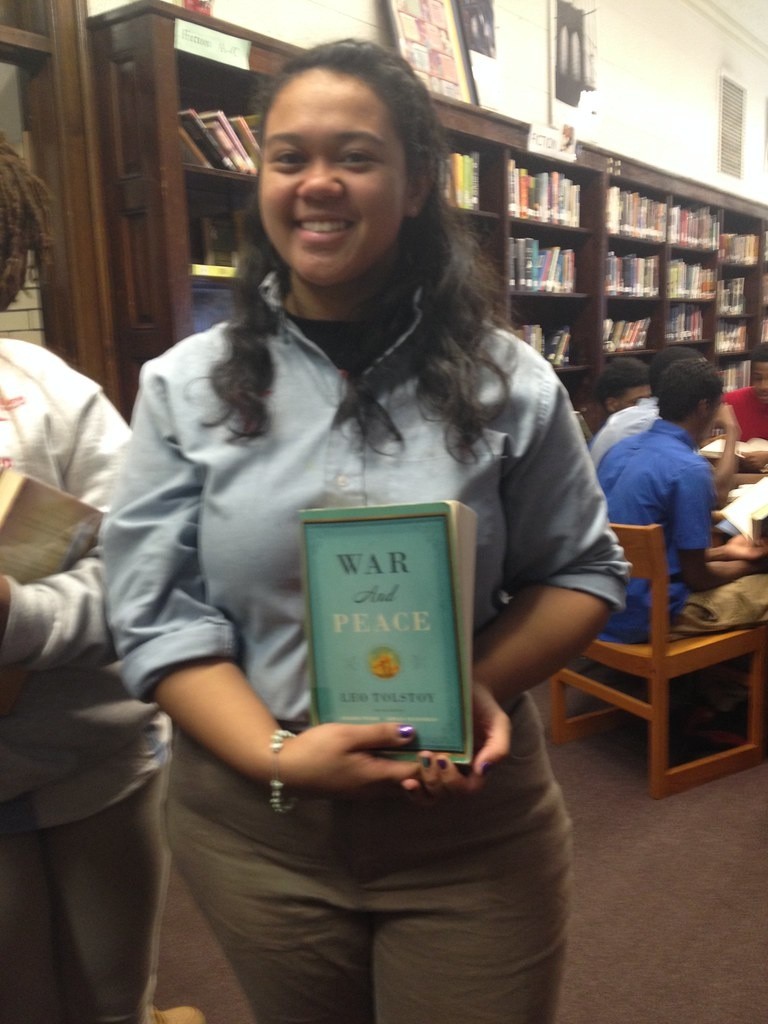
[0,0,768,440]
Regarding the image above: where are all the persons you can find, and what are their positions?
[98,37,632,1024]
[0,140,174,1024]
[589,348,739,505]
[723,342,768,470]
[600,358,652,414]
[593,356,768,749]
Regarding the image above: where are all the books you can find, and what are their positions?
[0,471,103,584]
[440,151,768,544]
[176,107,264,266]
[298,499,480,765]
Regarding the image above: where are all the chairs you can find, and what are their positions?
[549,523,768,802]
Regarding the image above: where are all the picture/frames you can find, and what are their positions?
[381,0,480,106]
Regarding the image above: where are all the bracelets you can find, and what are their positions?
[270,729,300,813]
